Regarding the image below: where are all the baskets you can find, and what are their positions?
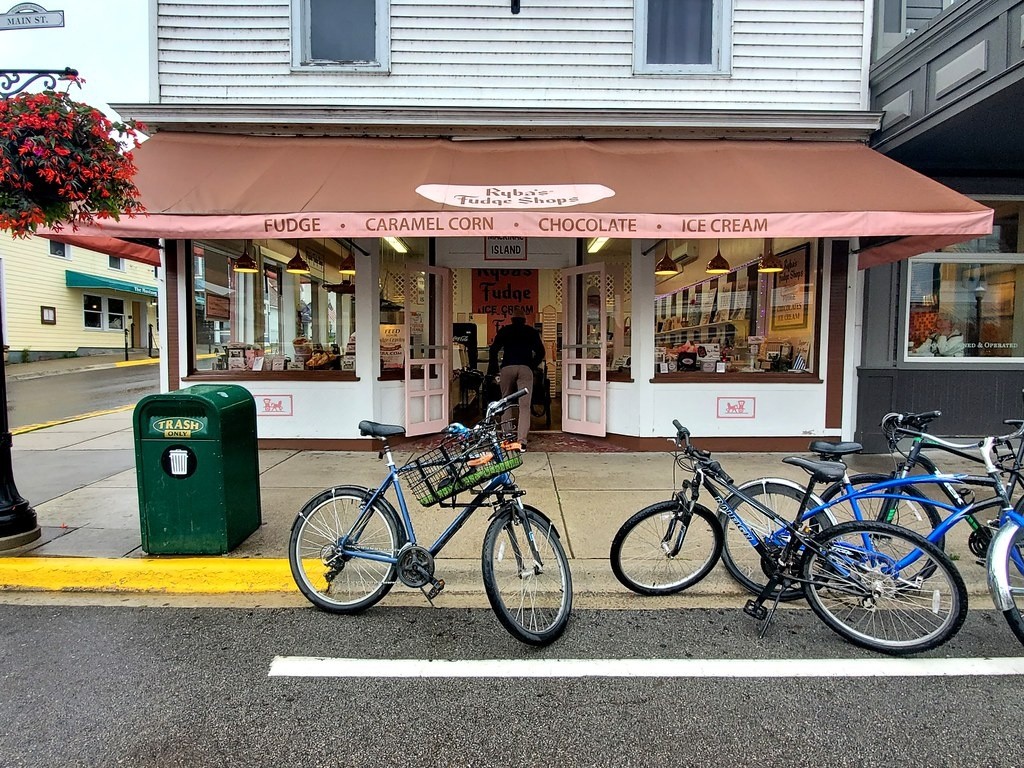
[400,419,522,507]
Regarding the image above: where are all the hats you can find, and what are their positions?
[512,310,526,318]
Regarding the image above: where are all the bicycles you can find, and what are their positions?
[811,411,1024,590]
[289,387,574,648]
[717,428,1024,646]
[610,419,968,653]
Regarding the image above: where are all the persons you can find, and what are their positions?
[488,310,546,452]
[911,311,964,358]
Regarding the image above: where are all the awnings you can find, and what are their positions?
[34,131,994,270]
[67,269,158,298]
[195,278,206,311]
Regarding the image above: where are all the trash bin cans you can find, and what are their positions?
[133,384,261,553]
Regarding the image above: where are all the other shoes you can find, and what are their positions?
[521,444,527,452]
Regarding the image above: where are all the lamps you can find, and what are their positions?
[233,240,260,272]
[757,238,783,272]
[706,238,730,274]
[286,239,311,273]
[339,238,356,275]
[654,240,678,275]
[587,237,610,254]
[383,236,411,253]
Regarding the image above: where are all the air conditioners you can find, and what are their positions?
[672,240,699,264]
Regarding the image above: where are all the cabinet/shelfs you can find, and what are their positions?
[656,319,750,349]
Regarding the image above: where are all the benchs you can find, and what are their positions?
[754,342,790,372]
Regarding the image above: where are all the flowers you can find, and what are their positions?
[0,72,149,239]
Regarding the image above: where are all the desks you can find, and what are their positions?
[453,347,469,369]
